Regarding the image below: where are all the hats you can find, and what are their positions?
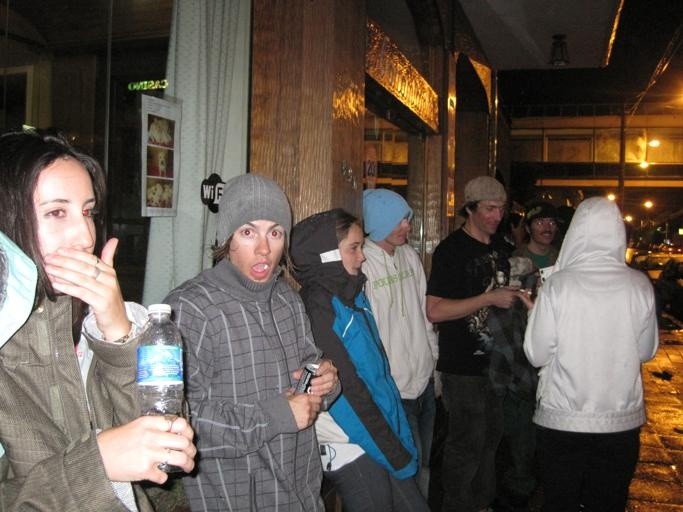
[362,187,414,241]
[524,202,561,220]
[216,173,291,253]
[456,175,509,217]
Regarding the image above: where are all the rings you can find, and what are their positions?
[93,266,101,280]
[164,448,172,466]
[161,414,180,433]
[93,256,99,267]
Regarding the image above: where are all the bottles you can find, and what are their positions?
[132,303,183,476]
[493,243,509,290]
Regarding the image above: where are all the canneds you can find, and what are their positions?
[293,363,320,396]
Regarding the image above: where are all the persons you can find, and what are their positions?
[523,196,659,510]
[490,202,575,511]
[287,206,433,510]
[426,176,521,511]
[0,125,200,511]
[358,186,439,483]
[158,173,343,511]
